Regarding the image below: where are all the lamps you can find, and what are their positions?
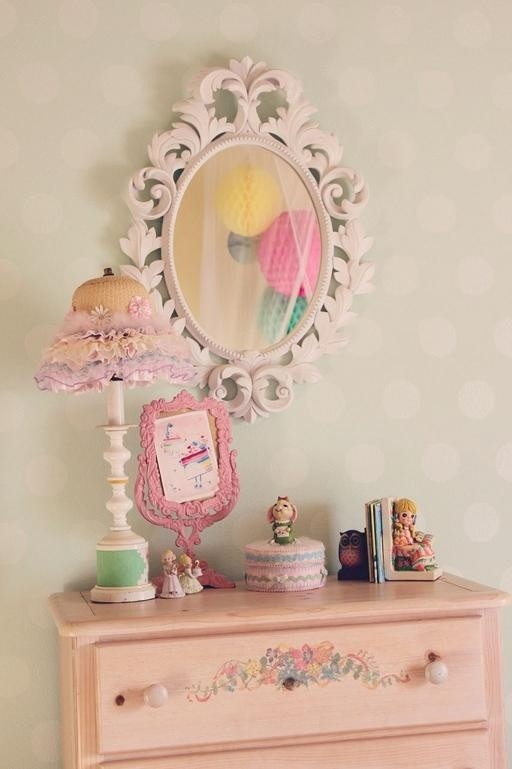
[32,267,198,606]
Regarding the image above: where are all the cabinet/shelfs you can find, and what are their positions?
[47,568,507,768]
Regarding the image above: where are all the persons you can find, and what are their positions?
[381,496,439,581]
[158,549,187,599]
[179,554,204,594]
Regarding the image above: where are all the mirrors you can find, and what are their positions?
[117,55,372,425]
